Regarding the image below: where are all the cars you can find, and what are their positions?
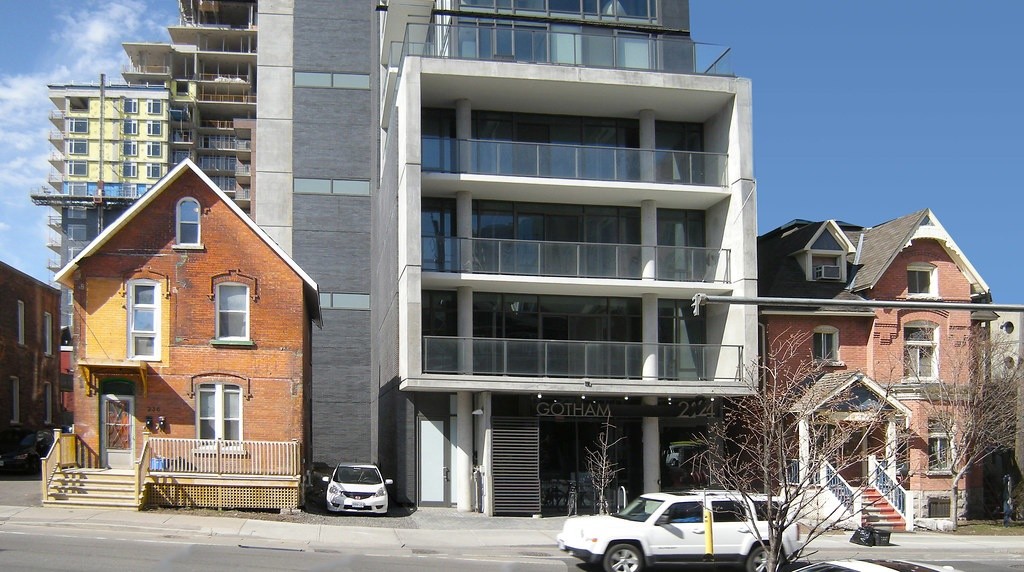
[0,422,70,477]
[312,461,333,489]
[322,461,394,517]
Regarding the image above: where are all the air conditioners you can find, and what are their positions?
[816,266,841,279]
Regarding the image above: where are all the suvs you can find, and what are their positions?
[556,489,806,572]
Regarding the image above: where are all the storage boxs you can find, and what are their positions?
[871,529,892,546]
